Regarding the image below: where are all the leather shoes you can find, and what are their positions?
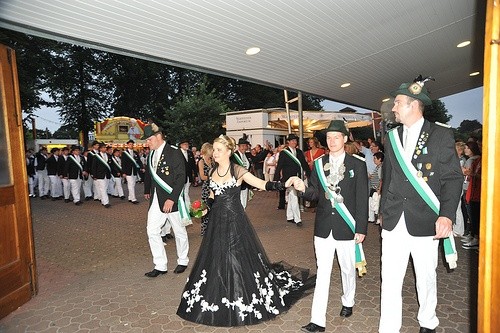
[301,323,325,333]
[297,222,303,226]
[174,264,187,273]
[340,306,352,316]
[30,196,125,208]
[287,219,295,223]
[419,327,437,333]
[162,236,168,242]
[145,269,168,277]
[166,233,174,239]
[129,200,132,202]
[132,201,139,204]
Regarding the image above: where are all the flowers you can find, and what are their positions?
[190,200,207,218]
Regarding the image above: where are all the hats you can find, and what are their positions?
[454,136,466,142]
[390,73,432,105]
[236,138,251,146]
[127,140,134,144]
[285,134,300,141]
[321,120,350,136]
[467,142,479,155]
[39,141,120,153]
[140,123,161,140]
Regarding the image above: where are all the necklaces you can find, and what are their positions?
[216,163,230,177]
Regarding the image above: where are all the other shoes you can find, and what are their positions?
[460,236,473,243]
[462,238,478,249]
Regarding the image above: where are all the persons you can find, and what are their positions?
[26,134,482,250]
[378,82,464,333]
[195,134,299,326]
[140,123,189,278]
[293,120,369,333]
